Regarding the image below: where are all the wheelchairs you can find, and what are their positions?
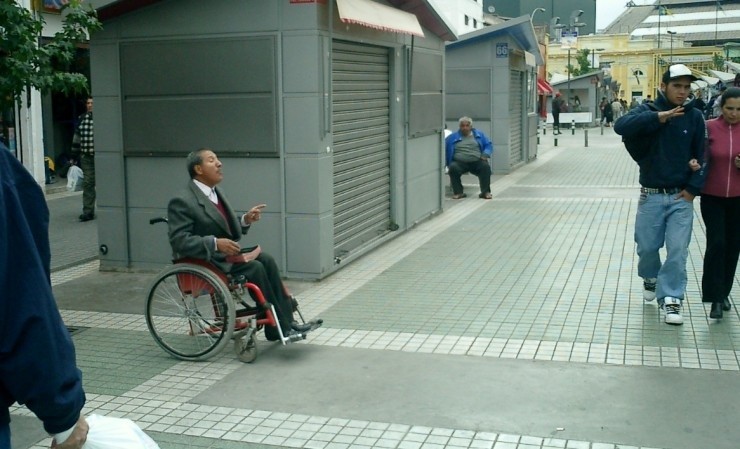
[144,216,324,363]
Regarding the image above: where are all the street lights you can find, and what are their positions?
[667,31,677,66]
[531,7,546,21]
[592,48,605,69]
[715,45,729,73]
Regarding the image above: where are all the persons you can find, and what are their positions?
[72,98,96,220]
[169,149,322,343]
[0,147,90,449]
[445,63,740,324]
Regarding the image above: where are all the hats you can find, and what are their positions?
[663,63,698,83]
[556,92,560,96]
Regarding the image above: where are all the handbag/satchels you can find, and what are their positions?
[56,413,161,449]
[67,165,84,192]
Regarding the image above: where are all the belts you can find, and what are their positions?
[640,187,677,194]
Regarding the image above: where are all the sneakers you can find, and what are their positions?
[663,297,683,324]
[642,277,656,301]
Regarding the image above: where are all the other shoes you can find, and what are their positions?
[293,319,324,332]
[79,214,94,220]
[723,298,731,311]
[710,302,723,319]
[481,193,492,199]
[267,328,306,341]
[454,192,464,199]
